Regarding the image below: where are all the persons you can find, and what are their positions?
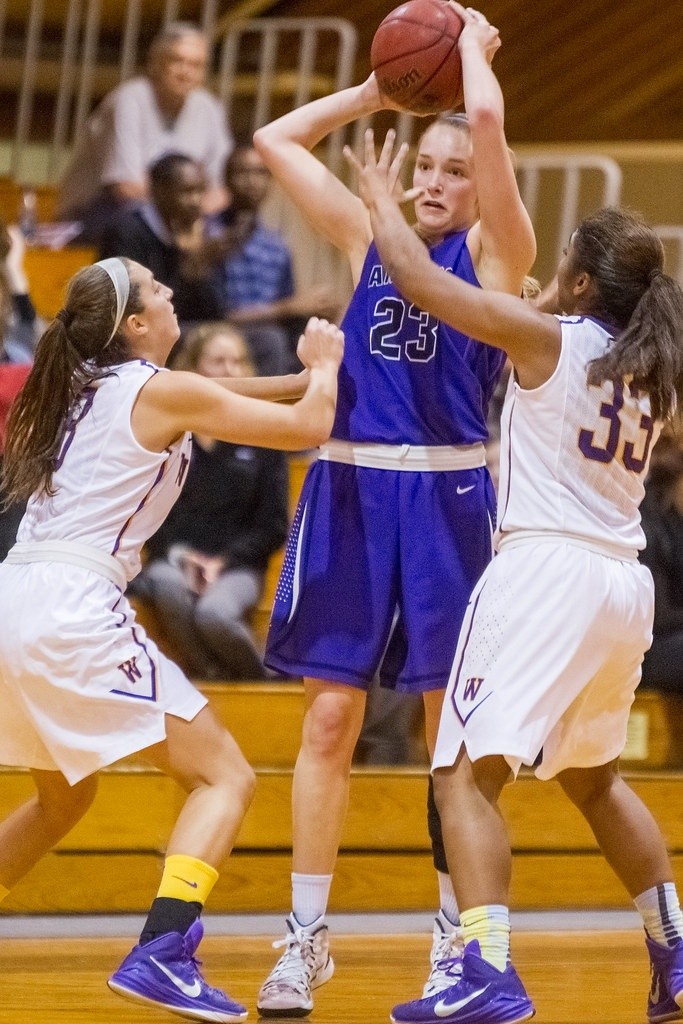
[0,256,345,1024]
[0,24,342,681]
[253,0,683,1024]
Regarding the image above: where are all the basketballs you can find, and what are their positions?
[368,1,475,117]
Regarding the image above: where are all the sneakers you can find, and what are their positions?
[107,916,249,1024]
[420,910,467,1000]
[255,909,334,1020]
[387,939,535,1024]
[644,937,683,1023]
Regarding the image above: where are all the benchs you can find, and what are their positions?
[0,181,683,909]
